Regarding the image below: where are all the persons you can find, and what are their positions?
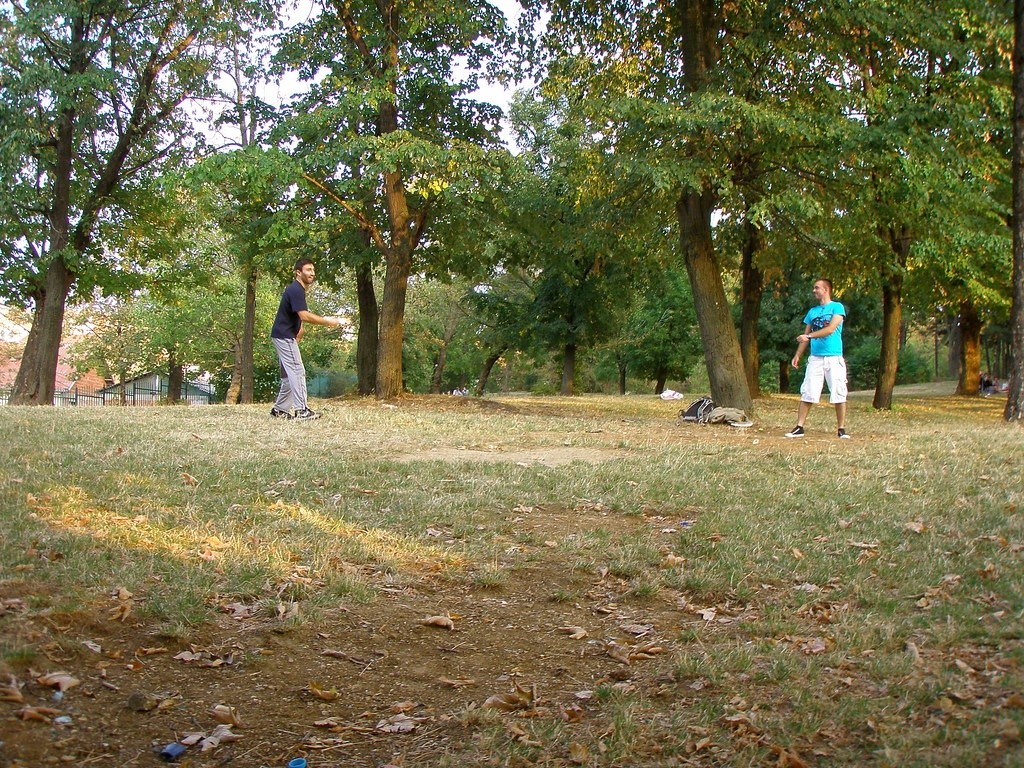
[784,278,851,439]
[978,370,1010,395]
[453,387,463,396]
[268,258,339,422]
[461,386,471,396]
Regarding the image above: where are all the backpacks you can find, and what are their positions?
[678,395,713,422]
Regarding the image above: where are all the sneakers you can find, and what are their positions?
[837,428,850,439]
[271,408,294,420]
[295,408,323,420]
[785,425,804,437]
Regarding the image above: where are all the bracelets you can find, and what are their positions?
[805,334,811,340]
